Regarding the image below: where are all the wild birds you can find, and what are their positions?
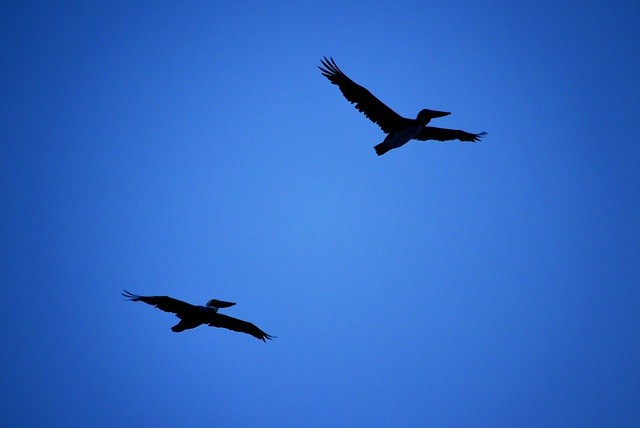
[318,55,489,156]
[120,289,278,343]
